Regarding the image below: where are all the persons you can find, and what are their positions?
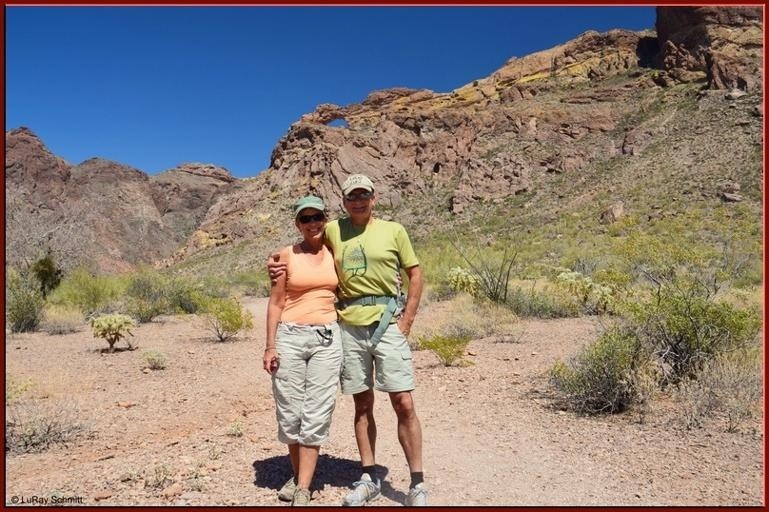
[266,172,430,507]
[260,196,404,508]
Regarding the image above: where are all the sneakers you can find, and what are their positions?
[342,473,384,507]
[291,487,311,506]
[404,482,426,506]
[277,476,298,501]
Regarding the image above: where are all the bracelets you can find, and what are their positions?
[264,346,276,352]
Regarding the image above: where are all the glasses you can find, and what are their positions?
[345,192,371,201]
[299,212,324,224]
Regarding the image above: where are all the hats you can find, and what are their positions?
[342,174,375,197]
[294,197,324,215]
